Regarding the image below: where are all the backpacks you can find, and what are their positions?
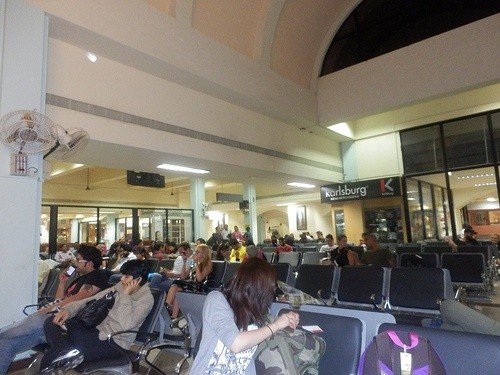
[253,324,326,375]
[358,331,448,375]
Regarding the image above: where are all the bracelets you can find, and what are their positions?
[266,324,274,335]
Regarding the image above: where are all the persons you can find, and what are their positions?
[0,221,500,375]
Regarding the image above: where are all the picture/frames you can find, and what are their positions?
[296,205,307,230]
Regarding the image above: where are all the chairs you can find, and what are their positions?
[23,239,500,375]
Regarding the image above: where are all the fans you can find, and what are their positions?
[43,123,89,181]
[0,109,56,176]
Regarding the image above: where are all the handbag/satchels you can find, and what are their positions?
[76,290,117,328]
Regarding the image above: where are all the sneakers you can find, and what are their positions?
[40,348,84,375]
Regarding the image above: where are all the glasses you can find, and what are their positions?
[76,259,89,263]
